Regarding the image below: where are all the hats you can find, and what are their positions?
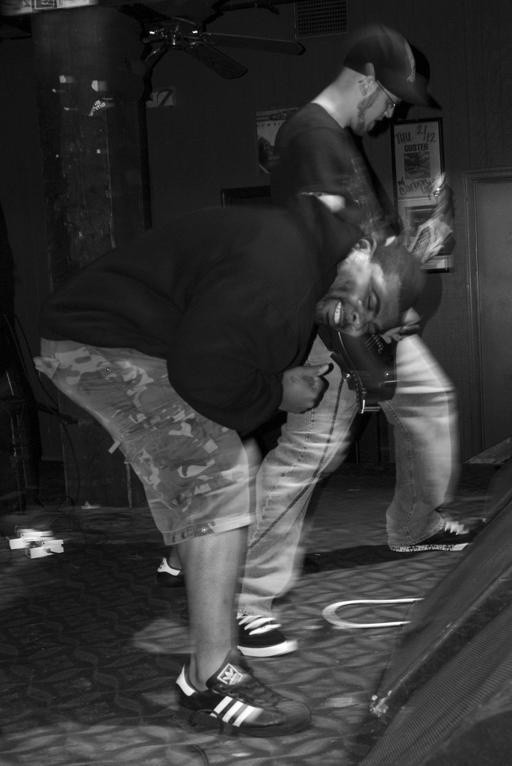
[343,27,425,106]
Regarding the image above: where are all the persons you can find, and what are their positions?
[236,28,487,657]
[32,186,425,737]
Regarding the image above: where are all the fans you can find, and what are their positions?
[97,0,304,81]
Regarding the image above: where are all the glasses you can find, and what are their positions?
[378,79,399,109]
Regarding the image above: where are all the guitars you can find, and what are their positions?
[318,174,451,405]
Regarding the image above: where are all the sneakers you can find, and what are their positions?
[233,612,299,657]
[155,554,183,587]
[388,515,473,553]
[175,648,311,737]
